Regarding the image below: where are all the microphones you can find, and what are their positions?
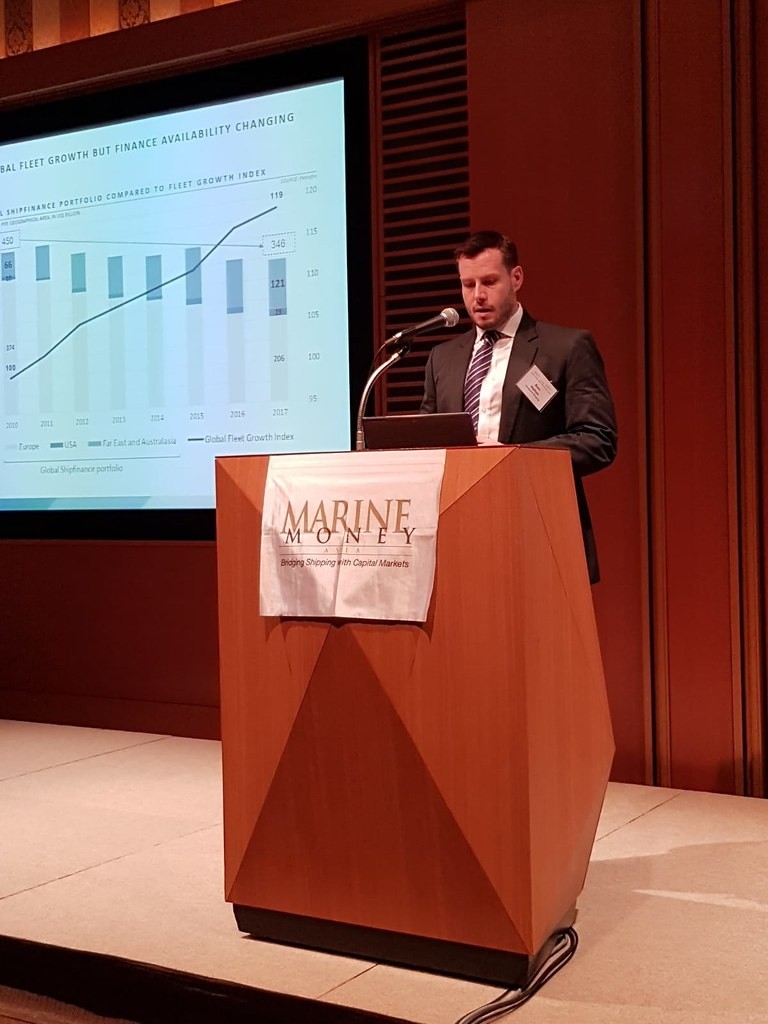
[385,307,461,347]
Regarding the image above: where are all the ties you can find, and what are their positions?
[462,329,505,436]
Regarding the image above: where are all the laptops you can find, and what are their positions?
[361,412,479,449]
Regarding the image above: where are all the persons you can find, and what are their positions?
[420,230,618,584]
